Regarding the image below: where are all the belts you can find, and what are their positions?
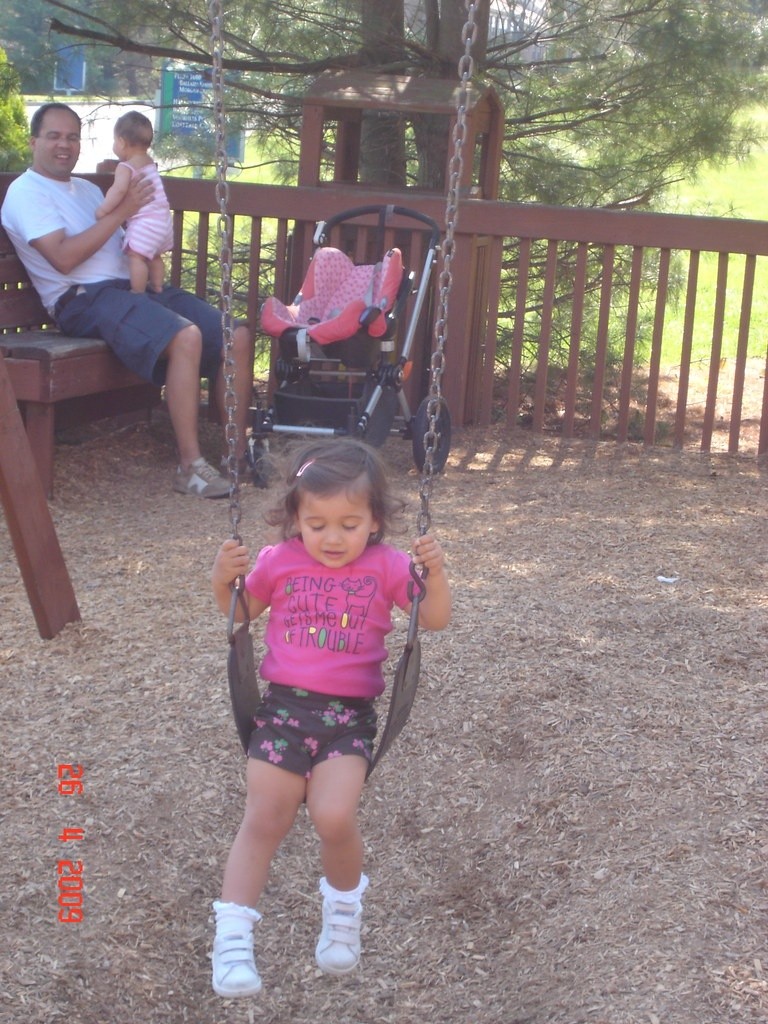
[54,284,78,315]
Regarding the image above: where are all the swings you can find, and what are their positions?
[211,0,487,785]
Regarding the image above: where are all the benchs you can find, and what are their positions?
[0,174,220,502]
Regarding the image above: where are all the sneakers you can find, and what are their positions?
[218,455,253,481]
[212,928,263,1000]
[174,457,234,497]
[314,899,364,975]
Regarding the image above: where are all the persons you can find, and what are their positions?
[95,112,174,293]
[211,436,450,996]
[0,103,278,498]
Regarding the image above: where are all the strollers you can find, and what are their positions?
[241,200,454,474]
